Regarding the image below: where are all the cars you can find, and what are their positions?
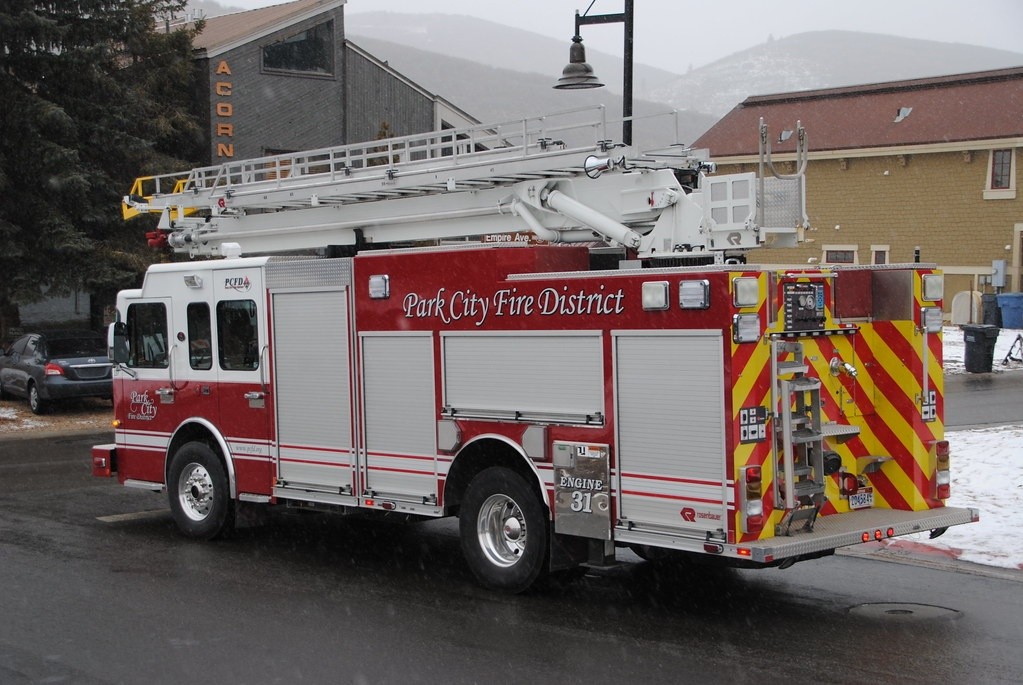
[0,328,115,415]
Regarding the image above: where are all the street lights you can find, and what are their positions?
[552,0,635,147]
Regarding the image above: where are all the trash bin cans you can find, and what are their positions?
[960,324,1000,373]
[996,293,1023,328]
[981,294,1002,328]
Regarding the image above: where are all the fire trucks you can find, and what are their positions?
[88,104,981,596]
